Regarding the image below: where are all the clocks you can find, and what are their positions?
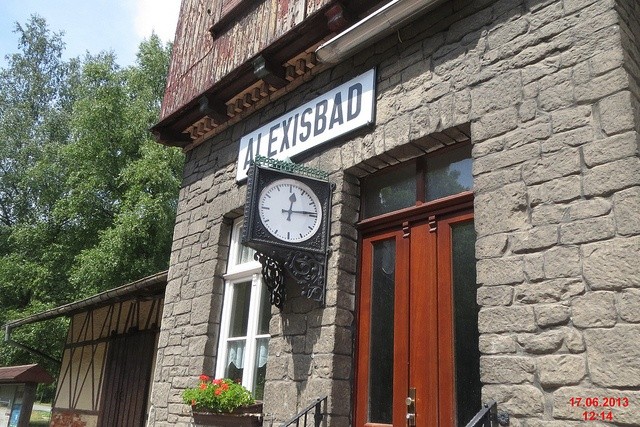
[239,163,329,307]
